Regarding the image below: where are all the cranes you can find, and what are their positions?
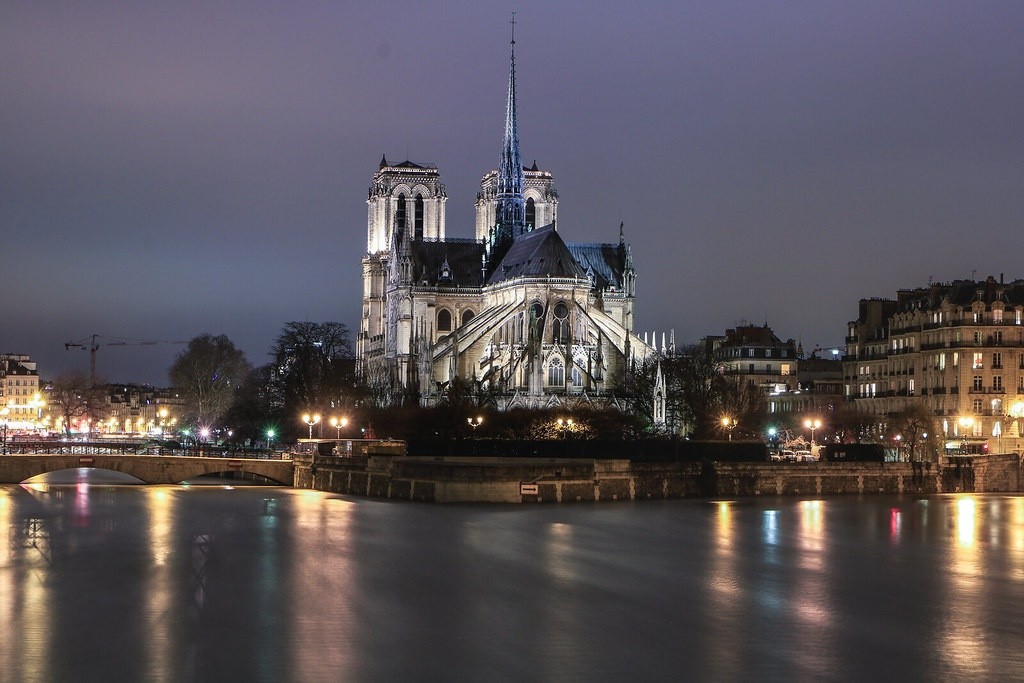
[64,334,192,388]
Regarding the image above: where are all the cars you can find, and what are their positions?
[770,449,814,463]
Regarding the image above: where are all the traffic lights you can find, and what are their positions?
[982,444,988,453]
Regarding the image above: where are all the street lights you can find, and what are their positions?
[184,429,190,448]
[721,417,739,441]
[329,415,348,438]
[959,416,974,454]
[467,416,482,438]
[302,414,321,438]
[806,419,820,462]
[268,430,274,459]
[201,429,210,442]
[558,418,572,439]
